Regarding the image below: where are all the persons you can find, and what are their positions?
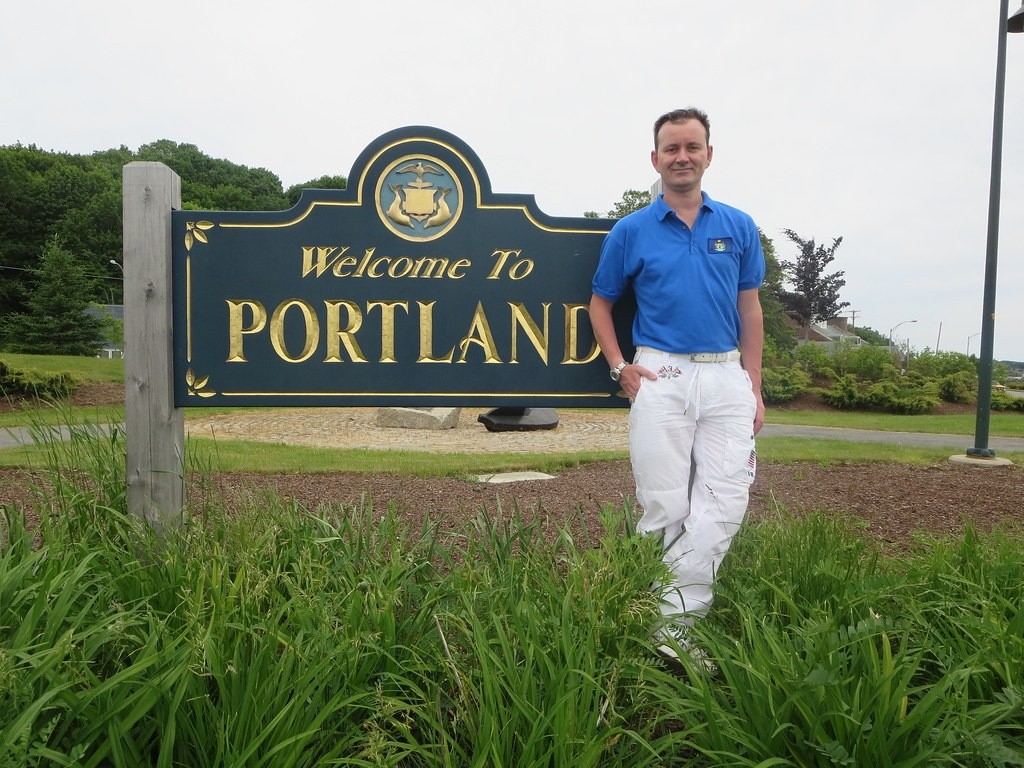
[589,109,765,674]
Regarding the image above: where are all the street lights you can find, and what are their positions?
[888,320,918,357]
[966,332,982,357]
[109,259,124,275]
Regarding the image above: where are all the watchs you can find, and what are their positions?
[610,362,627,382]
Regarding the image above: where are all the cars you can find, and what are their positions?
[991,379,1005,393]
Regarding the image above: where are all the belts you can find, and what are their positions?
[637,345,742,362]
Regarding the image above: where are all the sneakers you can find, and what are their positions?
[645,619,720,678]
